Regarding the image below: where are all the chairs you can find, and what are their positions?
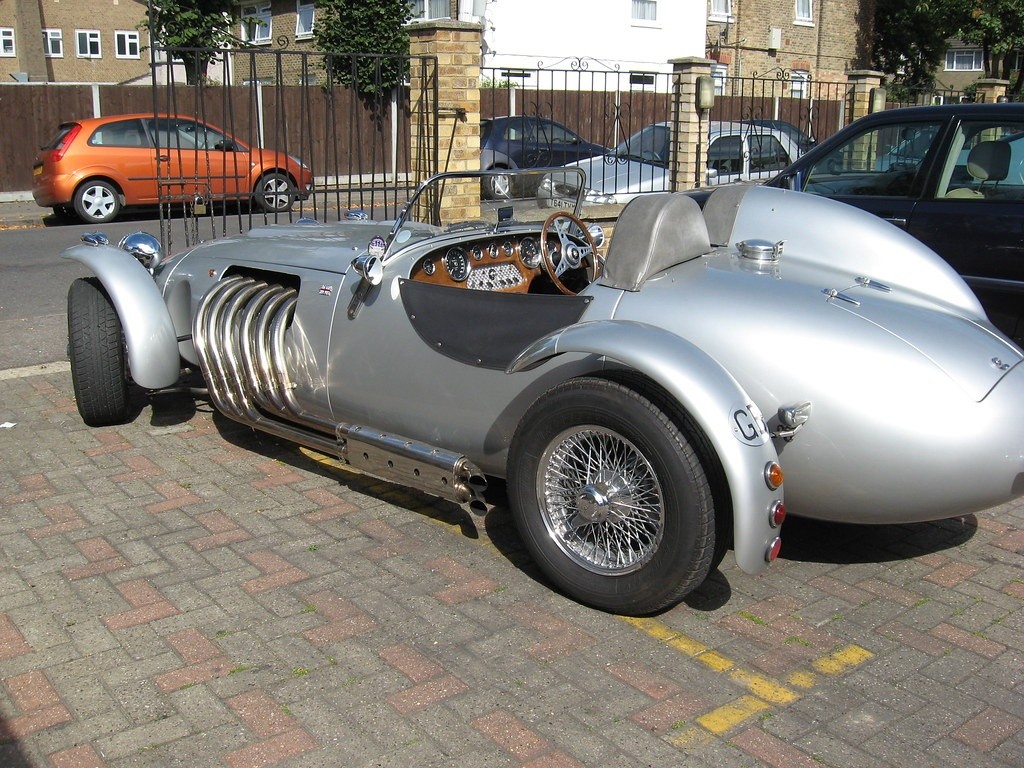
[944,140,1012,199]
[125,134,139,147]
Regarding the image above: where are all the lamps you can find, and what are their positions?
[869,88,886,115]
[695,77,714,109]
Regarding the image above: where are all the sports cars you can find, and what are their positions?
[57,177,1024,622]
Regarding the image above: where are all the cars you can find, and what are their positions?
[537,120,843,212]
[659,117,844,175]
[31,111,315,226]
[480,114,612,202]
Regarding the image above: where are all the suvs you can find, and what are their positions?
[759,101,1024,349]
